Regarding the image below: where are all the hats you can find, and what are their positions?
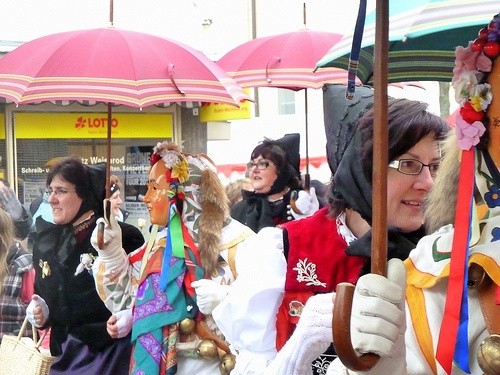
[83,162,106,204]
[322,83,395,176]
[276,133,302,179]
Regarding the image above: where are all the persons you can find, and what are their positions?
[0,177,57,350]
[27,156,145,375]
[224,177,255,208]
[215,83,449,375]
[230,133,330,233]
[88,144,257,375]
[327,14,500,375]
[105,175,129,222]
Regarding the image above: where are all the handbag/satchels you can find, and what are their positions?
[0,315,58,375]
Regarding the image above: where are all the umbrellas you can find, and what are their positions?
[0,23,257,250]
[314,0,500,85]
[216,25,425,216]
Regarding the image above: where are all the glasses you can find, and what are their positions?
[247,161,280,170]
[46,188,76,197]
[387,157,440,178]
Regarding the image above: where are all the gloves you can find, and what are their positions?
[0,187,23,221]
[347,258,407,375]
[90,199,124,263]
[191,279,227,315]
